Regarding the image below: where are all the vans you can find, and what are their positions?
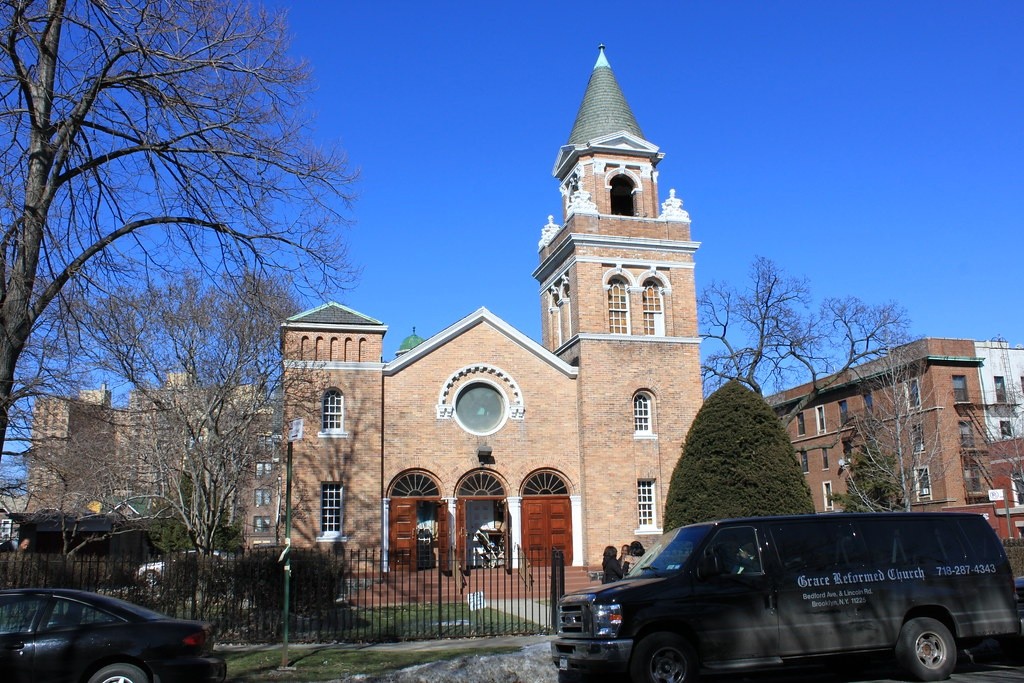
[550,511,1021,683]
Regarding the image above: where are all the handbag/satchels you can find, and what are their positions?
[602,571,607,583]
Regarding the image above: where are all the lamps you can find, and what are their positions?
[477,443,493,458]
[838,458,845,471]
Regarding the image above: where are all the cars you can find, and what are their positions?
[0,588,227,683]
[132,550,233,588]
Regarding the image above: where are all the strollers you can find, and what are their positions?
[473,532,505,569]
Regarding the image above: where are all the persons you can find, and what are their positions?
[622,541,645,576]
[602,546,622,584]
[15,538,34,552]
[617,545,630,570]
